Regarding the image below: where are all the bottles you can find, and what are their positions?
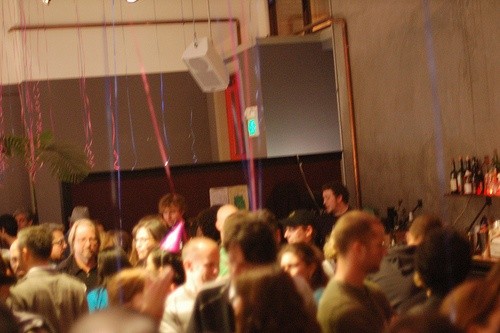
[449,152,500,196]
[477,216,489,256]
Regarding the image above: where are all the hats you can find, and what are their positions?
[280,209,318,226]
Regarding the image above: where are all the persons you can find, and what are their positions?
[368,215,499,333]
[323,184,351,219]
[0,192,389,333]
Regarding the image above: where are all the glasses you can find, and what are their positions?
[370,239,395,249]
